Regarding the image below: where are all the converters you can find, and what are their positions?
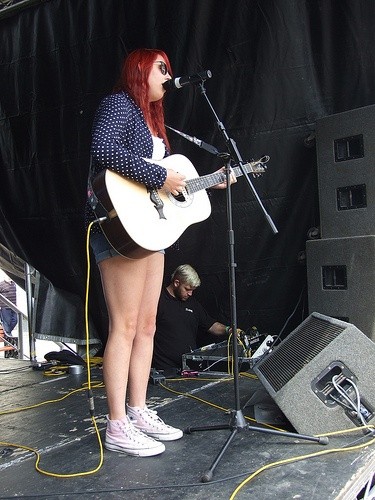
[150,375,166,384]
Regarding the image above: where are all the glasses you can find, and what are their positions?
[153,61,171,76]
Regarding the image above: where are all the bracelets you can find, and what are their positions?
[225,326,231,334]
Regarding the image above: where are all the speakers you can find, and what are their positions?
[306,236,375,343]
[314,105,375,239]
[253,311,375,436]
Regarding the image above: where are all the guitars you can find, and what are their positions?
[89,152,268,260]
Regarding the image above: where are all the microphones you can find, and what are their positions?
[163,68,212,91]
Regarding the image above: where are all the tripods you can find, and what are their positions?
[187,79,327,486]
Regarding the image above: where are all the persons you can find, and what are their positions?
[151,264,244,370]
[0,273,17,336]
[86,49,238,457]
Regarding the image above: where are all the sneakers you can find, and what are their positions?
[126,403,183,441]
[105,414,165,456]
